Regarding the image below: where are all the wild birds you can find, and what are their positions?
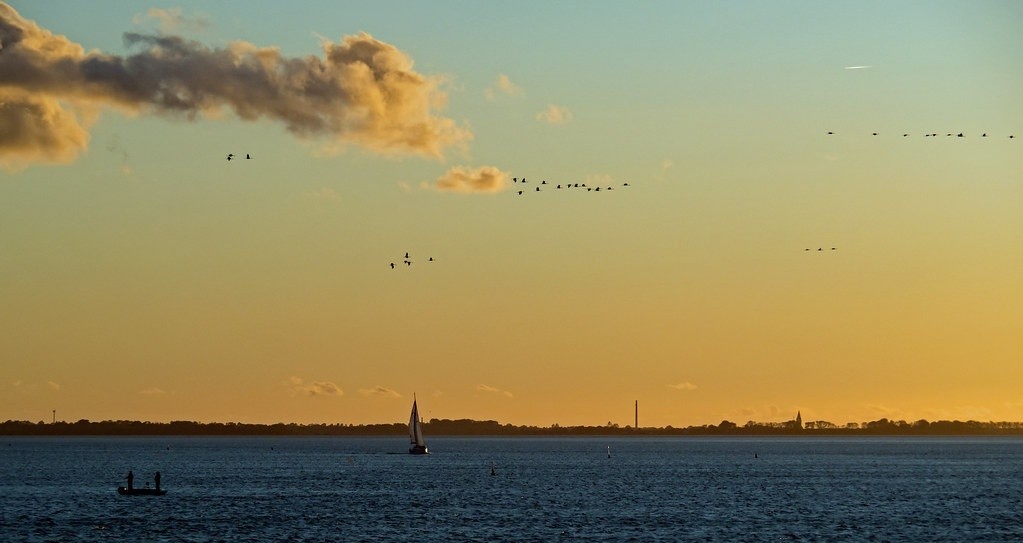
[512,176,631,195]
[388,252,435,270]
[946,134,951,136]
[226,154,253,161]
[872,132,879,135]
[831,248,838,250]
[1009,135,1015,138]
[902,134,908,137]
[957,133,966,138]
[805,248,810,251]
[828,132,836,134]
[981,133,988,137]
[926,134,936,136]
[817,248,823,251]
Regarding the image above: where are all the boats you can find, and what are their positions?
[118,486,168,496]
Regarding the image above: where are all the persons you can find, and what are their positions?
[124,471,133,490]
[154,471,160,490]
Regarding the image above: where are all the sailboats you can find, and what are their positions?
[408,392,429,455]
